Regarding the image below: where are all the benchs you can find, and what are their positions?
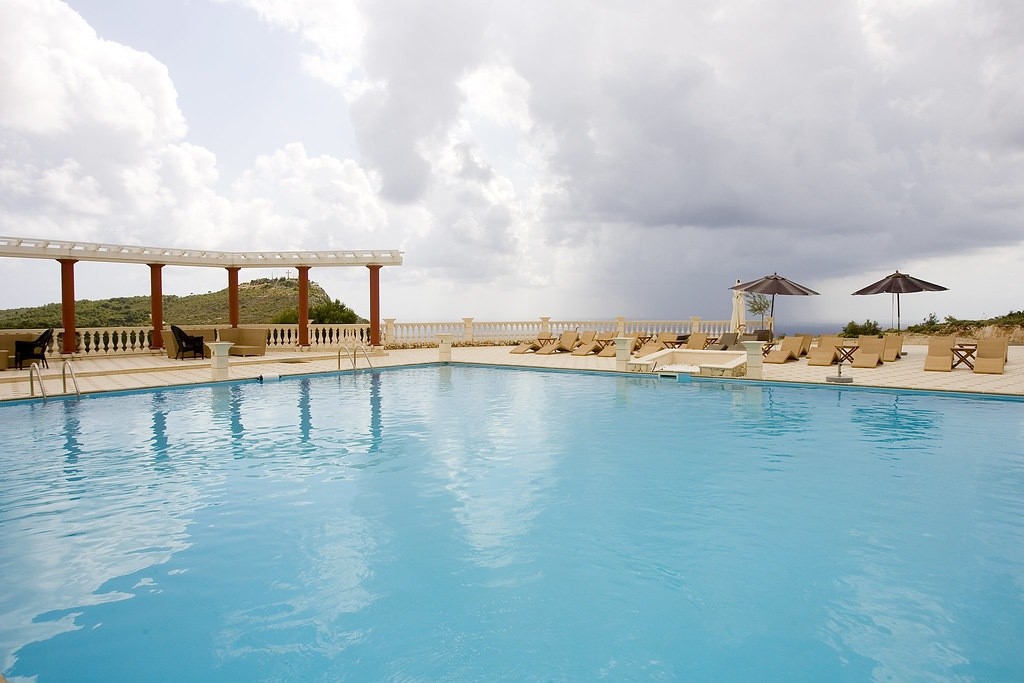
[161,329,215,358]
[0,333,43,368]
[219,327,267,357]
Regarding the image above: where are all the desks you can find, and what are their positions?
[704,337,717,350]
[636,337,652,352]
[950,347,976,370]
[834,345,858,364]
[958,343,977,360]
[663,340,684,350]
[762,343,777,357]
[595,340,614,349]
[538,338,557,353]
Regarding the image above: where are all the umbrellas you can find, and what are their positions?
[730,278,746,339]
[850,270,950,336]
[728,272,821,343]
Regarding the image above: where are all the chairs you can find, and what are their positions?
[169,325,204,361]
[15,328,53,370]
[924,337,956,371]
[974,336,1009,375]
[510,330,903,369]
